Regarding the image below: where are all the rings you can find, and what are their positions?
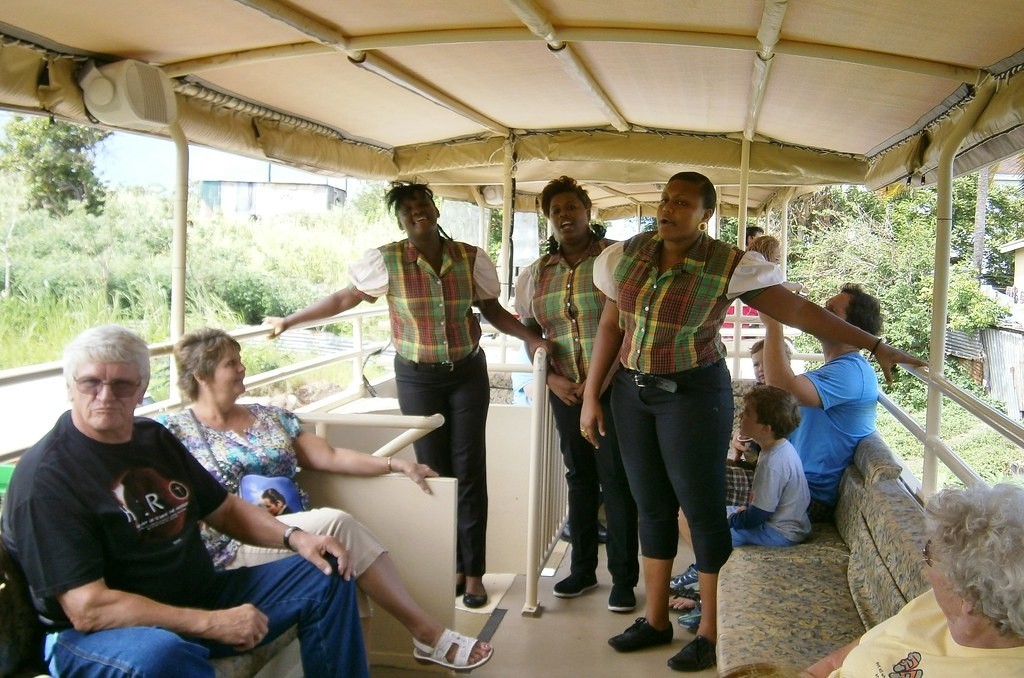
[580,429,588,437]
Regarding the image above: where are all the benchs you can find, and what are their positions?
[0,363,935,678]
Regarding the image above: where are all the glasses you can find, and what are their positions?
[74,377,141,399]
[922,538,945,568]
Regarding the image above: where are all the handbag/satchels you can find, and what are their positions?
[235,475,303,516]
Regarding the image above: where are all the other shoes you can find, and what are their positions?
[608,582,636,612]
[454,585,464,597]
[553,571,599,597]
[463,586,487,608]
[561,519,609,544]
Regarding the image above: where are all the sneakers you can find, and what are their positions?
[668,566,700,590]
[607,613,674,652]
[677,599,703,631]
[667,634,715,671]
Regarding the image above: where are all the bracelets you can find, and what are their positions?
[284,526,304,554]
[869,339,883,357]
[388,456,393,473]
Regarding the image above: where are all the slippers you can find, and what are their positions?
[668,586,704,611]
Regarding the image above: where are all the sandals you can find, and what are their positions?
[411,623,493,671]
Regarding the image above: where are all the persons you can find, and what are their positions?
[580,170,930,672]
[261,175,554,608]
[670,384,811,629]
[0,326,369,678]
[669,282,883,615]
[514,175,639,613]
[262,488,294,516]
[721,233,810,339]
[793,481,1024,678]
[154,326,494,672]
[746,227,764,247]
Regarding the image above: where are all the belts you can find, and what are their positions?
[625,359,719,393]
[396,346,481,373]
